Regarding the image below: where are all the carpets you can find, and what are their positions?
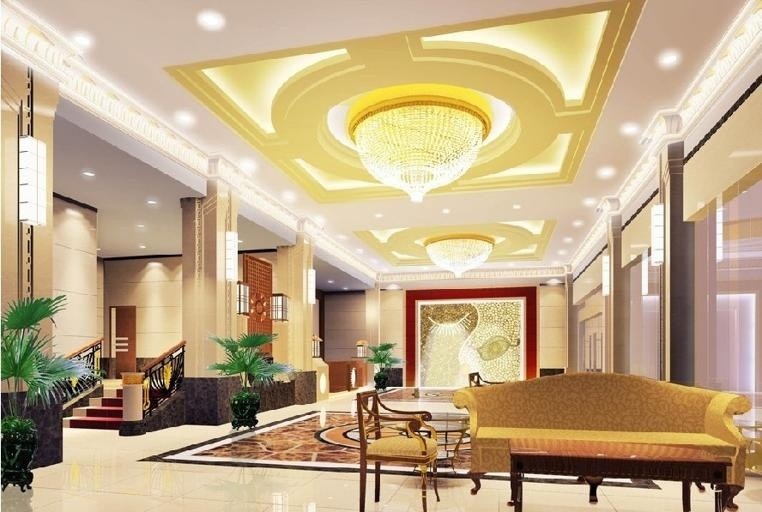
[70,389,163,429]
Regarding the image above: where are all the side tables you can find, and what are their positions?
[414,414,470,485]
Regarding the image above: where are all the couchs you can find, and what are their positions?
[453,371,753,512]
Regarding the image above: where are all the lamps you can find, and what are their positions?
[269,293,289,321]
[18,134,47,226]
[424,233,496,277]
[226,231,239,282]
[651,205,664,267]
[236,281,250,316]
[344,83,493,203]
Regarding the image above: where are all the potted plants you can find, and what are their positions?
[367,342,402,392]
[206,332,299,430]
[2,294,102,492]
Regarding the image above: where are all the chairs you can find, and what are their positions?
[357,390,440,512]
